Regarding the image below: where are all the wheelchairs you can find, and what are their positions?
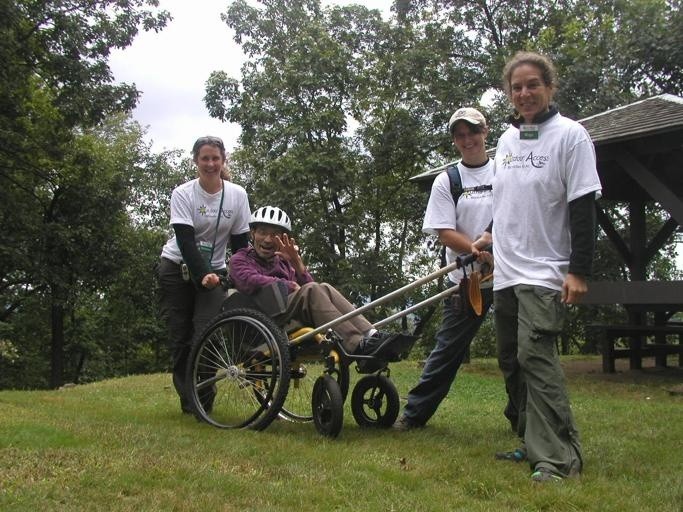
[185,244,413,439]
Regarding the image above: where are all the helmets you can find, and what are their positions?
[248,205,292,232]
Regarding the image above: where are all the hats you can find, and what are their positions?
[448,107,486,133]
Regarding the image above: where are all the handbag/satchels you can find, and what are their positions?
[192,260,215,291]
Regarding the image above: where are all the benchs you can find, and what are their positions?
[584,318,683,374]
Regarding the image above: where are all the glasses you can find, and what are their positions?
[197,136,223,145]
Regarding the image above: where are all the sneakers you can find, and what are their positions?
[495,447,528,462]
[391,414,418,431]
[531,467,562,482]
[357,331,412,362]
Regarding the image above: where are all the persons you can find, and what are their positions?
[227,207,408,374]
[470,50,602,484]
[159,136,252,422]
[387,106,523,433]
